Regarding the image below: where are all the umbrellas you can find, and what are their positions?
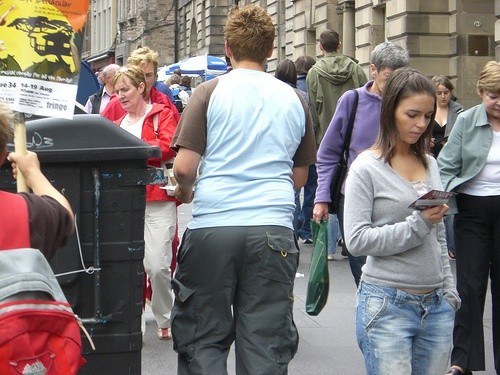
[165,53,228,80]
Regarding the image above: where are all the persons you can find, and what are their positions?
[154,74,174,104]
[0,105,76,253]
[170,7,317,375]
[425,73,466,157]
[103,47,181,123]
[85,63,119,115]
[305,30,366,260]
[313,43,408,289]
[163,70,198,112]
[342,68,461,375]
[112,65,179,339]
[436,61,500,375]
[274,55,320,245]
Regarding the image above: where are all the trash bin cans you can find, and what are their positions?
[1,113,163,375]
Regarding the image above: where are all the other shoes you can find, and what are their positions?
[338,237,344,246]
[328,254,334,261]
[303,238,313,244]
[157,326,173,340]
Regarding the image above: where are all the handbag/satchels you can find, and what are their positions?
[306,218,330,316]
[329,89,359,201]
[161,155,196,196]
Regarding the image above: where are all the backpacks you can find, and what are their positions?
[0,248,96,375]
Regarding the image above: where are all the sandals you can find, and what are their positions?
[446,368,473,375]
